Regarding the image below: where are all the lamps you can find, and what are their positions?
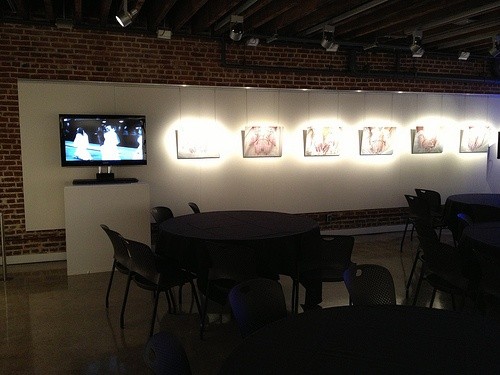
[489,40,500,58]
[321,26,336,48]
[116,0,140,28]
[229,14,245,42]
[456,49,471,61]
[410,31,424,58]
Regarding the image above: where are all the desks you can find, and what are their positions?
[157,209,321,323]
[444,193,500,241]
[216,304,500,375]
[459,220,500,297]
[63,180,151,276]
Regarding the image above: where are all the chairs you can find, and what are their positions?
[143,331,192,375]
[101,202,356,339]
[398,187,486,315]
[344,263,396,306]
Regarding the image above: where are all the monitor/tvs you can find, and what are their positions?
[59,114,147,166]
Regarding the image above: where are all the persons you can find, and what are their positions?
[73,122,145,160]
